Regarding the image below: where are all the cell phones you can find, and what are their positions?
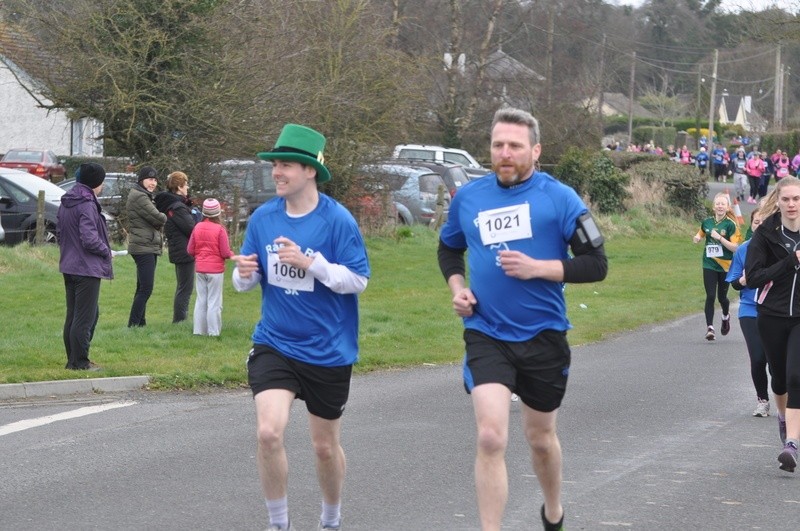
[576,211,605,249]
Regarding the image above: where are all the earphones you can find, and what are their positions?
[776,202,779,206]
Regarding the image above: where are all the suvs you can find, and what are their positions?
[206,160,276,223]
[349,143,492,226]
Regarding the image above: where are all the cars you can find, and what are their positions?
[55,173,139,217]
[0,166,120,248]
[0,149,68,184]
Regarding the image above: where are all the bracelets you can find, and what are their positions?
[719,235,722,242]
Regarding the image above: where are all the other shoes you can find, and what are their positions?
[740,193,744,200]
[541,504,564,531]
[81,362,101,371]
[265,520,295,531]
[747,196,757,204]
[317,517,342,531]
[737,194,741,201]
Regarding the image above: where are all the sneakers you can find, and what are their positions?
[778,444,798,473]
[776,412,789,444]
[706,328,715,341]
[753,398,771,417]
[721,313,729,335]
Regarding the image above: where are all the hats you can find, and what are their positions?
[201,198,222,217]
[138,167,159,183]
[257,124,331,183]
[75,163,105,189]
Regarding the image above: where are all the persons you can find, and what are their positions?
[744,174,800,473]
[724,208,789,417]
[230,122,372,530]
[187,197,234,337]
[153,171,198,325]
[437,108,609,531]
[59,159,70,179]
[692,192,740,341]
[606,128,800,205]
[56,163,114,371]
[125,165,167,328]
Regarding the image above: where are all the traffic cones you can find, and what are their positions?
[724,187,731,207]
[734,197,745,225]
